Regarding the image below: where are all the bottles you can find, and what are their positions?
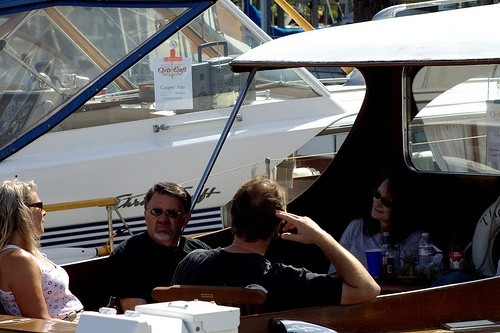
[381,232,395,276]
[107,296,120,314]
[419,233,433,270]
[450,243,464,272]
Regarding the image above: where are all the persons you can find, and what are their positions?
[172,178,381,318]
[327,171,443,275]
[0,178,84,321]
[105,182,212,315]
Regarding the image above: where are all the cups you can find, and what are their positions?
[365,249,382,277]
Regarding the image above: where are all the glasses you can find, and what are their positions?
[147,207,183,218]
[26,202,43,211]
[373,190,392,208]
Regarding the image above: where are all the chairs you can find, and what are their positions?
[151,285,269,316]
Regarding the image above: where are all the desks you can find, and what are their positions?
[374,277,423,295]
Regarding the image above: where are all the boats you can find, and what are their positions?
[0,0,500,251]
